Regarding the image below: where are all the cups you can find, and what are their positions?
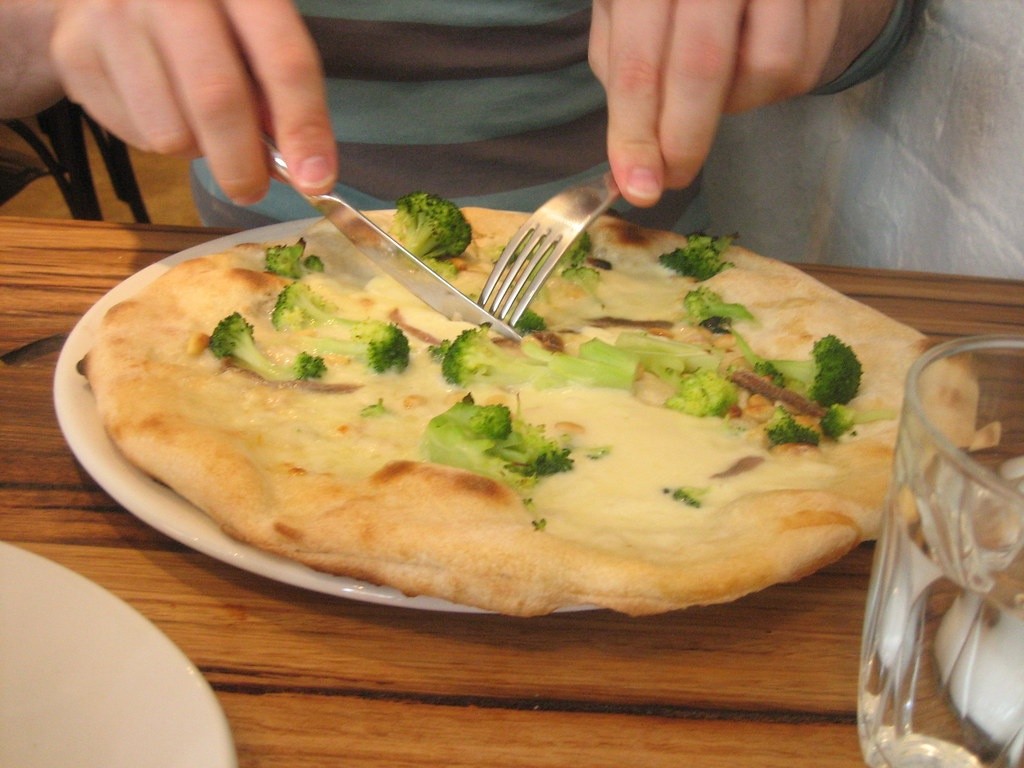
[856,332,1024,768]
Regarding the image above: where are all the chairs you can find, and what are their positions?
[0,100,149,223]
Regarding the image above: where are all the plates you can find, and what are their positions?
[51,216,607,614]
[0,539,238,768]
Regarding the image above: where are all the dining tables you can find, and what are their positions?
[0,213,1024,768]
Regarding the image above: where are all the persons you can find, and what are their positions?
[0,1,911,256]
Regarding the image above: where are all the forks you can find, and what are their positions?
[476,171,624,328]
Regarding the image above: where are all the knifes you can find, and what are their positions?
[265,135,525,344]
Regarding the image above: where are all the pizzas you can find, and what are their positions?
[77,192,978,617]
